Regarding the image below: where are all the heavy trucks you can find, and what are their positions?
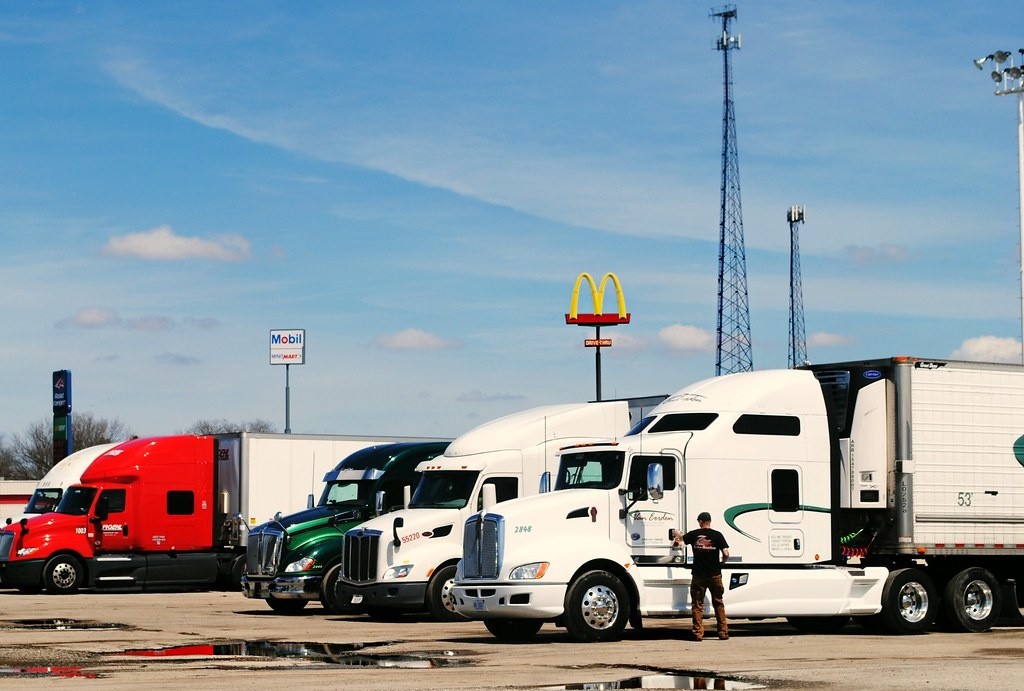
[448,358,1023,643]
[0,432,454,596]
[240,395,671,623]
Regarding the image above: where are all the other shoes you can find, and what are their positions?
[688,632,703,641]
[719,634,729,640]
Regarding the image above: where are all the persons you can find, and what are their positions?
[672,512,729,640]
